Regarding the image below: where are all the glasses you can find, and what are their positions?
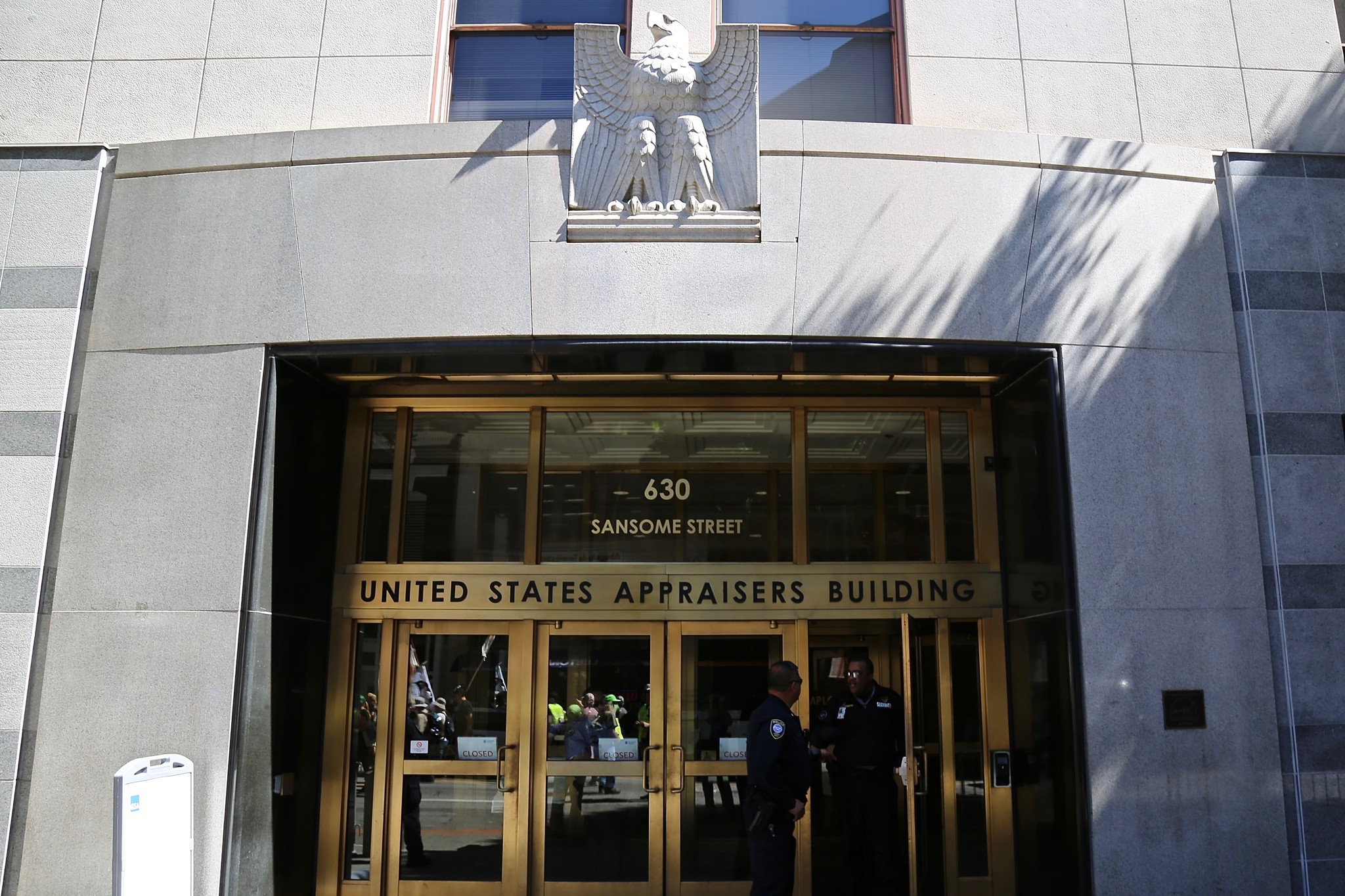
[788,679,803,685]
[843,669,862,678]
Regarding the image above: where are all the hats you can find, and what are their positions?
[567,704,581,717]
[453,683,466,695]
[617,696,624,702]
[581,692,594,702]
[359,695,366,703]
[414,697,428,707]
[432,697,446,710]
[604,694,620,702]
[644,684,650,691]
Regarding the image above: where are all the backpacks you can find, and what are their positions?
[359,709,376,744]
[588,710,611,747]
[437,712,454,748]
[418,709,445,746]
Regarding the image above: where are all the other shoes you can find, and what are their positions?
[599,786,621,795]
[406,853,432,867]
[588,781,598,786]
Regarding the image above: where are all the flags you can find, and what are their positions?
[481,635,496,661]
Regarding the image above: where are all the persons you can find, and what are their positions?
[812,657,906,896]
[353,684,475,869]
[497,682,748,825]
[742,661,838,896]
